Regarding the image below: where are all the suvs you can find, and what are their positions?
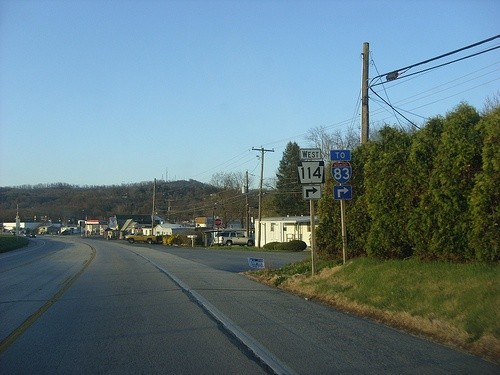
[213,230,254,247]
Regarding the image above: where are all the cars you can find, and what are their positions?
[125,232,156,245]
[23,232,37,238]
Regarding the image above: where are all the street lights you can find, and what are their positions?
[256,154,264,248]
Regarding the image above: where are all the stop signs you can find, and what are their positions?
[214,219,222,227]
